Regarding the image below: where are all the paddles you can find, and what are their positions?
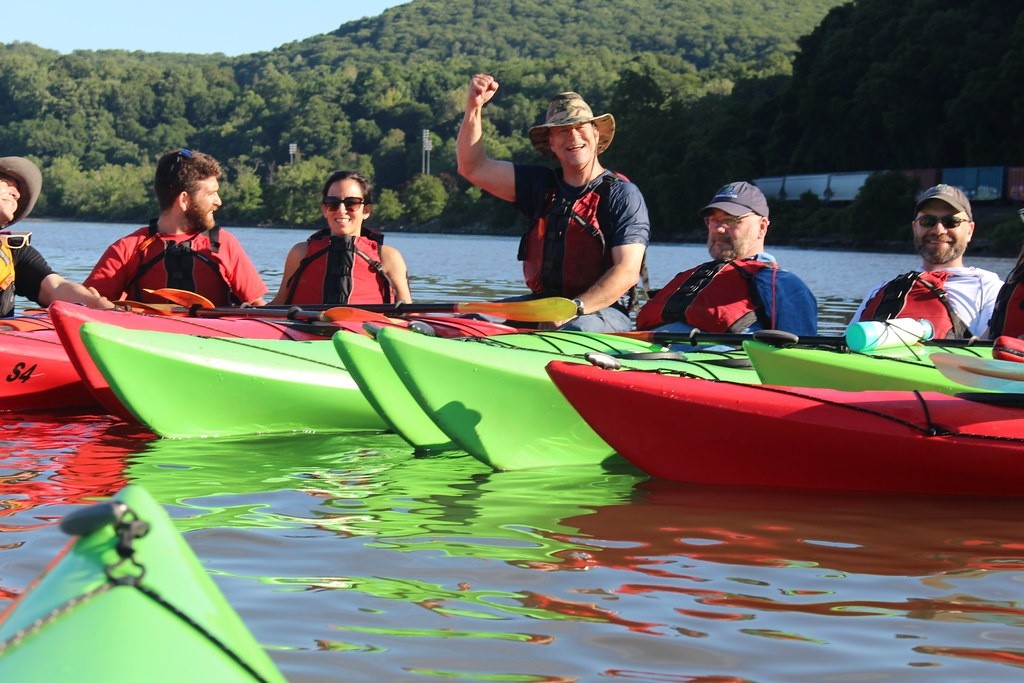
[142,288,578,322]
[112,301,408,325]
[516,328,799,348]
[930,352,1024,394]
[797,335,994,347]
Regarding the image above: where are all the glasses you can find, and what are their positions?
[914,214,972,229]
[705,213,757,229]
[169,149,192,190]
[1,230,33,248]
[322,196,366,212]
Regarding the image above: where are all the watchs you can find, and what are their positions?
[572,298,584,315]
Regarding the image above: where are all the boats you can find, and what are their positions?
[1,299,1023,504]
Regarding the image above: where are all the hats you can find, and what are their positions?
[700,181,769,219]
[0,156,42,229]
[917,184,973,221]
[528,92,616,159]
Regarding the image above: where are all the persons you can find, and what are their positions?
[635,183,819,336]
[990,208,1024,341]
[84,149,267,307]
[846,184,1001,339]
[1,156,115,318]
[268,170,412,304]
[455,75,649,331]
